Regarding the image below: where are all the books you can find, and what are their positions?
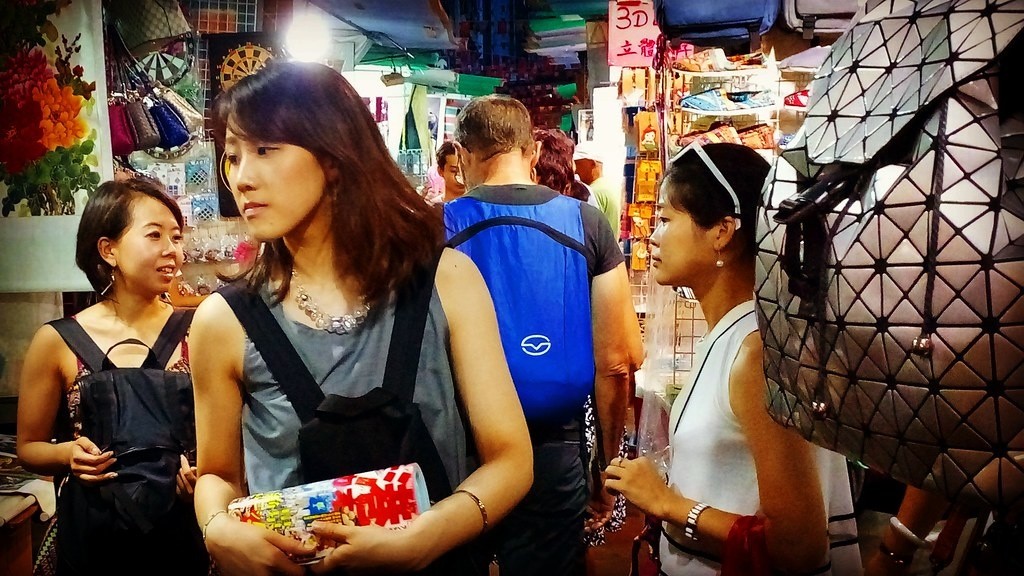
[227,462,433,567]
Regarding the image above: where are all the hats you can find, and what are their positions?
[572,141,604,162]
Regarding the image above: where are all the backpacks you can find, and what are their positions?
[213,248,490,576]
[44,309,209,576]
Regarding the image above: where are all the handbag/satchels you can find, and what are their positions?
[104,0,204,158]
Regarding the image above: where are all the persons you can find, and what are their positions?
[602,137,865,576]
[867,446,1024,576]
[189,59,536,576]
[15,179,249,576]
[430,92,653,576]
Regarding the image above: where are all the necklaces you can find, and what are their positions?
[285,258,375,336]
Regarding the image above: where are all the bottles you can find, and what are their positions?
[398,149,422,175]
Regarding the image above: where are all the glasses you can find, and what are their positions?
[454,156,464,186]
[667,140,741,230]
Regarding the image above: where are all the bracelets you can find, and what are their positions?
[684,502,713,546]
[203,510,233,556]
[452,490,488,536]
